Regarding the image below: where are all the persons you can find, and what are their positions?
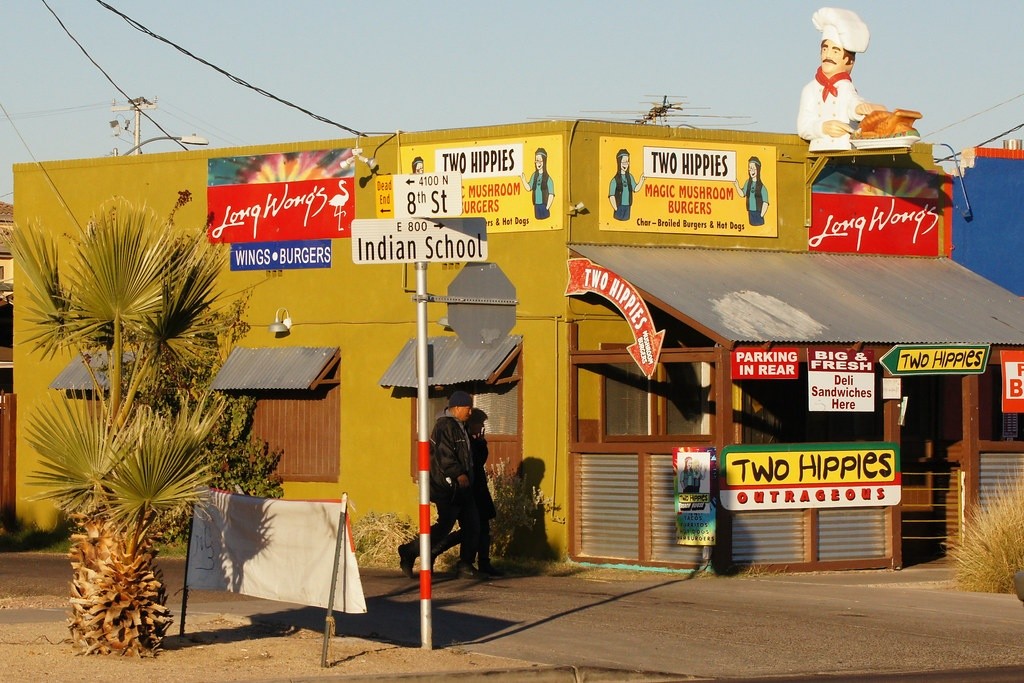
[796,5,885,152]
[397,391,502,580]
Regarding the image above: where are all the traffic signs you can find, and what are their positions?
[349,215,490,264]
[392,171,463,219]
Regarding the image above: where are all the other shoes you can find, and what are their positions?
[456,560,488,579]
[398,543,420,579]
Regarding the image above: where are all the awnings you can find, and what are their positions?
[210,346,339,389]
[375,335,525,387]
[50,345,146,393]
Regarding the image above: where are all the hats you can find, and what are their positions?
[444,391,473,415]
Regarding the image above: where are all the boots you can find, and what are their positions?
[430,531,461,578]
[478,535,504,579]
